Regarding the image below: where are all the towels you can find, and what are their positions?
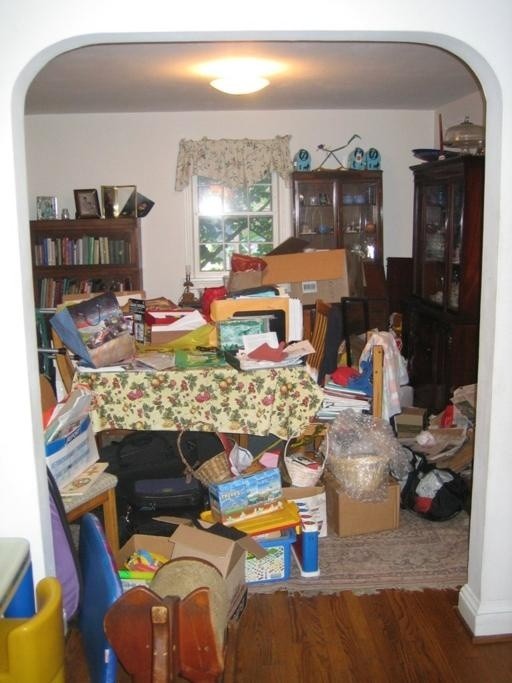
[359,331,410,421]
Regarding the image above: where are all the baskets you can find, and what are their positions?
[284,423,329,487]
[178,421,233,485]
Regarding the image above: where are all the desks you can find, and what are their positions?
[1,536,35,616]
[77,358,313,488]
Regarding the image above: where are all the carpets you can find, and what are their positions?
[241,433,469,599]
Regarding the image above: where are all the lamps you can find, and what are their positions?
[183,265,194,303]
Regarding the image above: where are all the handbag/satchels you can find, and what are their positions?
[50,291,136,368]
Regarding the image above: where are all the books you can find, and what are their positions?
[30,231,131,310]
[318,372,477,475]
[59,460,107,496]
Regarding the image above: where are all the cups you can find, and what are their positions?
[299,224,312,234]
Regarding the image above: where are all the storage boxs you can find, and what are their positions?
[139,309,206,347]
[316,462,403,539]
[45,415,101,488]
[110,470,328,634]
[257,250,361,302]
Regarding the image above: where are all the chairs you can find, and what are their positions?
[38,371,121,565]
[50,327,74,395]
[2,576,69,682]
[307,299,336,377]
[296,328,404,452]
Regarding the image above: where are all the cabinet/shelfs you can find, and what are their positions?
[28,218,143,305]
[400,291,480,415]
[297,167,381,260]
[409,154,484,309]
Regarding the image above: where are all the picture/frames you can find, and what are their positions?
[36,194,57,218]
[74,189,101,217]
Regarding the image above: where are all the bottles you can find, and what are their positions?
[310,191,328,205]
[365,185,373,204]
[62,208,69,219]
